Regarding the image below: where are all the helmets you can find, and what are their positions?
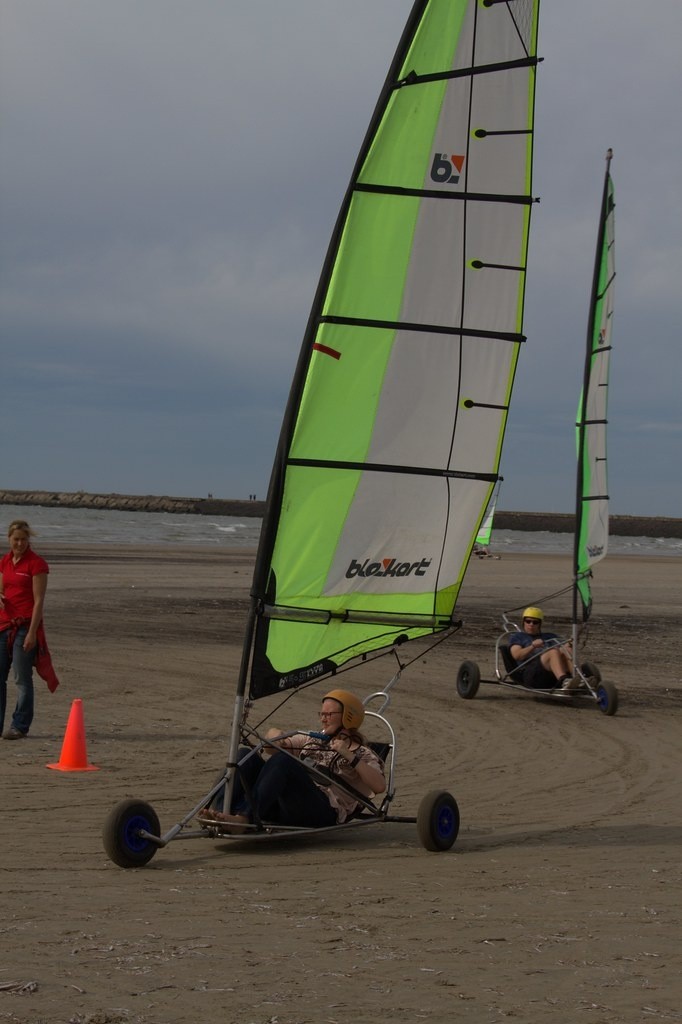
[322,690,364,731]
[522,606,544,620]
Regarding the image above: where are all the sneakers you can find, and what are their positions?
[2,728,27,740]
[562,675,581,694]
[578,677,596,693]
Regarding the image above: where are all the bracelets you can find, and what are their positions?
[351,755,360,767]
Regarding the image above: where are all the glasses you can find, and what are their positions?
[318,712,342,718]
[523,620,540,625]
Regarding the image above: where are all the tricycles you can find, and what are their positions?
[98,0,543,870]
[456,148,620,715]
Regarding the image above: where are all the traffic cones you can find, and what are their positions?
[46,698,100,771]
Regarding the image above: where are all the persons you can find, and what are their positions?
[208,493,212,498]
[198,689,386,835]
[0,519,49,739]
[249,493,257,500]
[510,607,597,695]
[473,544,492,557]
